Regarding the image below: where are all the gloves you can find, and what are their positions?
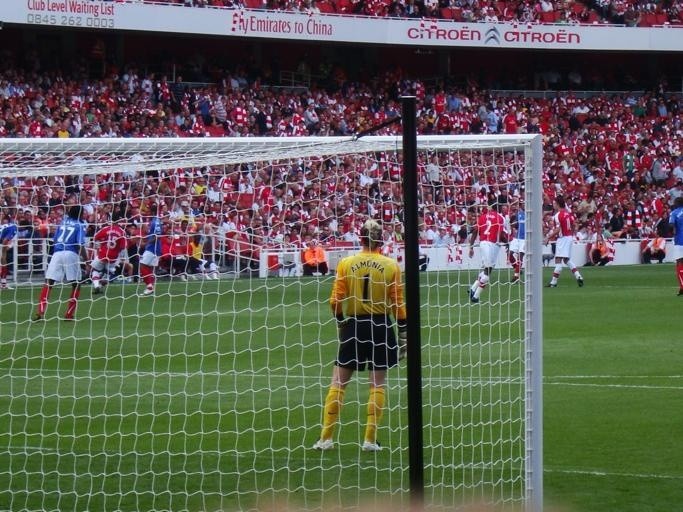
[397,341,410,360]
[336,320,346,339]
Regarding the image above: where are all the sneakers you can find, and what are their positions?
[362,441,384,452]
[312,437,335,451]
[139,285,155,297]
[509,275,586,289]
[32,317,45,323]
[91,286,104,295]
[0,282,15,290]
[466,288,483,304]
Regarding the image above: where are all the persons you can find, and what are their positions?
[465,201,509,304]
[310,220,406,452]
[543,195,585,289]
[30,205,87,321]
[138,203,162,297]
[90,213,126,295]
[504,201,526,286]
[663,196,682,297]
[1,0,683,282]
